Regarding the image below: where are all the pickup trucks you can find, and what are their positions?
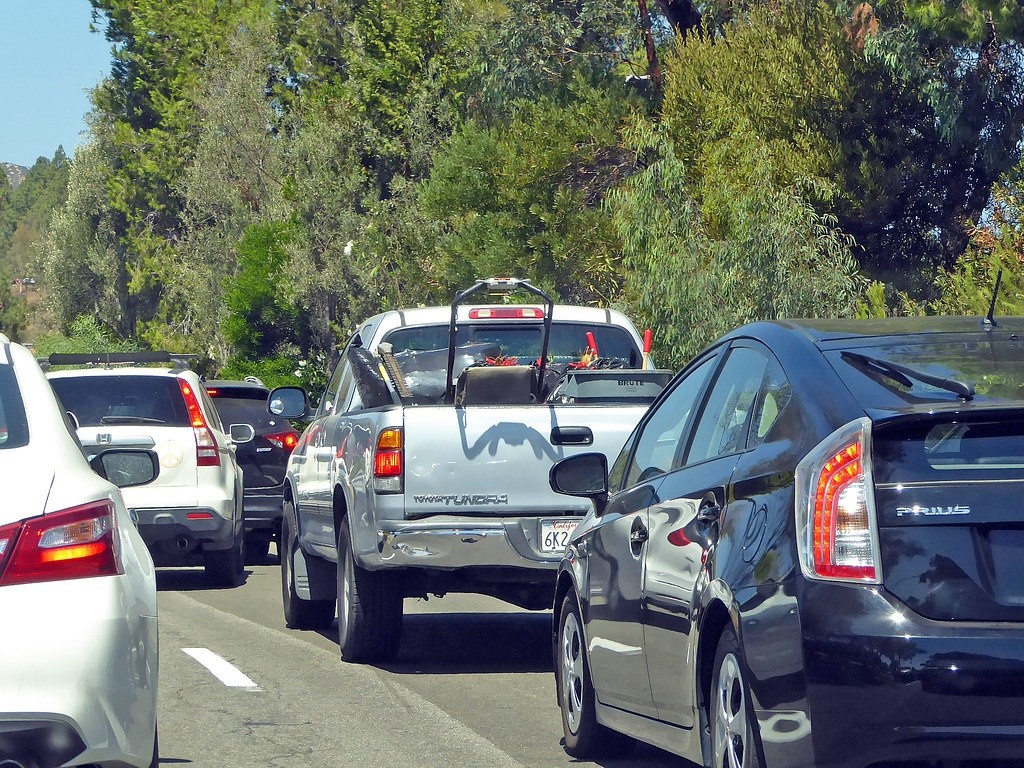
[266,276,679,665]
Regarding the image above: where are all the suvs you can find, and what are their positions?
[33,351,258,588]
[202,376,303,562]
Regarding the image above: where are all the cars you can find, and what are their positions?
[0,329,161,767]
[548,270,1024,768]
